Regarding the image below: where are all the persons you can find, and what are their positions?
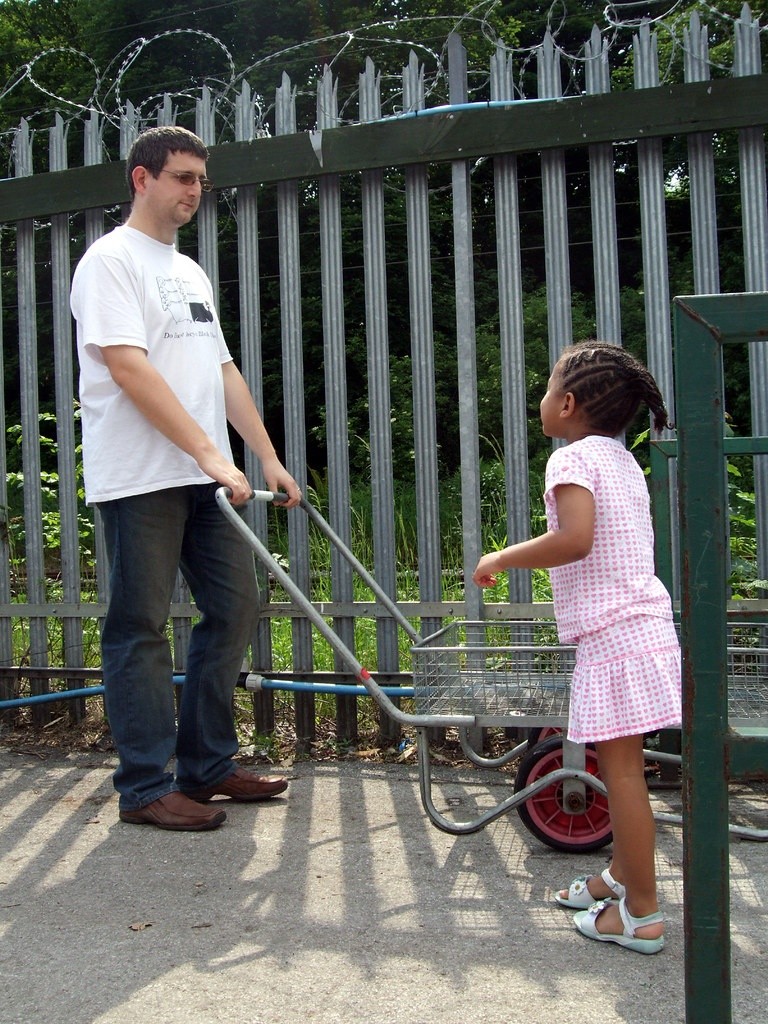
[469,340,684,953]
[69,125,303,829]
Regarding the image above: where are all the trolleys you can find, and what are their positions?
[213,483,768,855]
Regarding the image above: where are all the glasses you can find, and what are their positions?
[145,167,214,192]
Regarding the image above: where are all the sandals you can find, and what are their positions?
[573,897,665,955]
[554,868,626,909]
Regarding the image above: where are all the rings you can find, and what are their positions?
[298,488,301,491]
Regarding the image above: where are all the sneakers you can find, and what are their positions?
[183,764,287,803]
[119,791,228,832]
